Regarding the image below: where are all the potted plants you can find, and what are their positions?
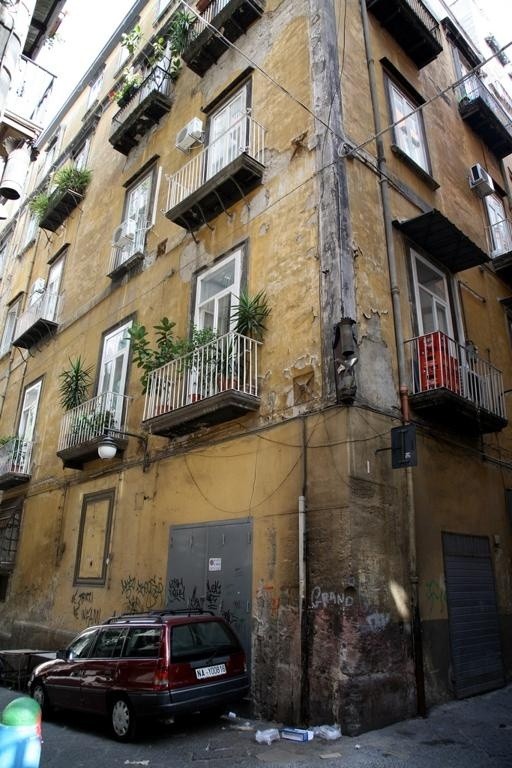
[210,288,272,392]
[123,318,176,416]
[178,319,224,406]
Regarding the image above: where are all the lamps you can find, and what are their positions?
[98,428,148,461]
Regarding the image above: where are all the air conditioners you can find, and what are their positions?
[28,292,42,307]
[470,163,495,194]
[112,218,136,249]
[176,117,203,151]
[28,277,44,297]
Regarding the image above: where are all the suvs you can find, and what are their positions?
[28,608,251,743]
